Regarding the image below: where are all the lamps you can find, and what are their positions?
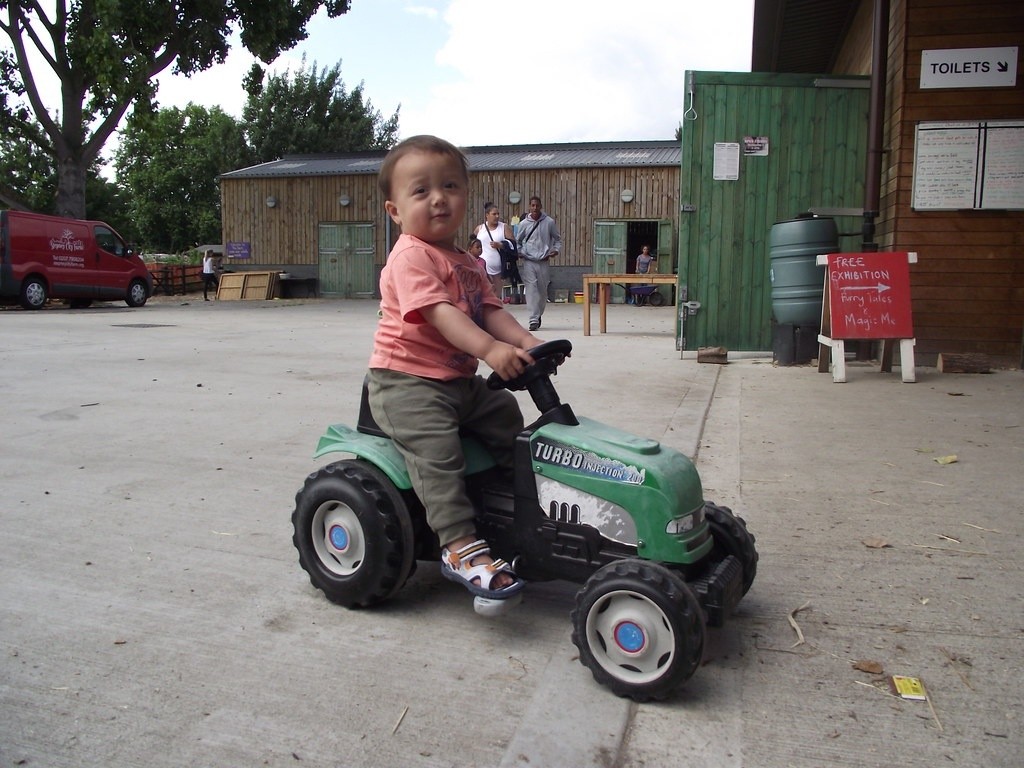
[266,196,276,207]
[509,191,520,204]
[339,195,350,205]
[621,189,633,202]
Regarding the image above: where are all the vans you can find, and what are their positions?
[0,209,153,308]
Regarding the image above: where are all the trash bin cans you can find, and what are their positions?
[768,212,842,366]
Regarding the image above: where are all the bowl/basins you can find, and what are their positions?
[574,296,583,303]
[279,274,290,279]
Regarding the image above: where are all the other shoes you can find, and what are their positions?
[538,316,541,328]
[530,323,539,330]
[205,299,210,301]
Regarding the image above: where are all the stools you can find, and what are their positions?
[517,283,526,294]
[502,284,512,301]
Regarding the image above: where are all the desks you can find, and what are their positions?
[148,270,171,296]
[281,278,317,298]
[583,273,678,336]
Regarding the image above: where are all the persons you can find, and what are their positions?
[202,249,219,301]
[515,197,562,331]
[466,233,486,270]
[635,246,652,306]
[473,202,515,298]
[366,134,566,599]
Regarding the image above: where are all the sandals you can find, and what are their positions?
[441,539,526,599]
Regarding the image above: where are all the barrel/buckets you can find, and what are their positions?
[597,284,610,303]
[770,213,839,327]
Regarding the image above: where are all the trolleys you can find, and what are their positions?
[614,283,658,307]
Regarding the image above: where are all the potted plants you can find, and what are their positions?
[279,270,291,279]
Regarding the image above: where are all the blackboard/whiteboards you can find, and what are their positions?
[828,252,913,340]
